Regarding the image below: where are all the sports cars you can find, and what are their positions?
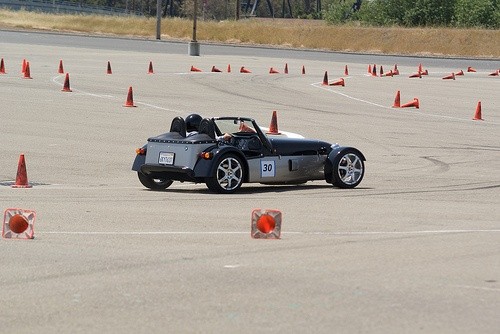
[132,116,366,194]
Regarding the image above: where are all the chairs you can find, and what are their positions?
[170,116,186,138]
[198,117,215,139]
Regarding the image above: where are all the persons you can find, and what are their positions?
[185,114,232,141]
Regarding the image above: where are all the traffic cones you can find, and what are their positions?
[391,90,420,109]
[322,65,349,86]
[2,209,36,239]
[11,154,32,187]
[60,72,73,92]
[251,209,283,239]
[368,62,477,80]
[487,68,500,76]
[0,57,7,74]
[107,61,112,74]
[472,101,485,120]
[147,61,155,74]
[190,63,252,74]
[22,59,33,79]
[269,63,306,75]
[58,59,65,74]
[122,86,137,107]
[238,111,282,135]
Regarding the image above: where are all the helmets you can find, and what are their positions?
[185,114,203,133]
